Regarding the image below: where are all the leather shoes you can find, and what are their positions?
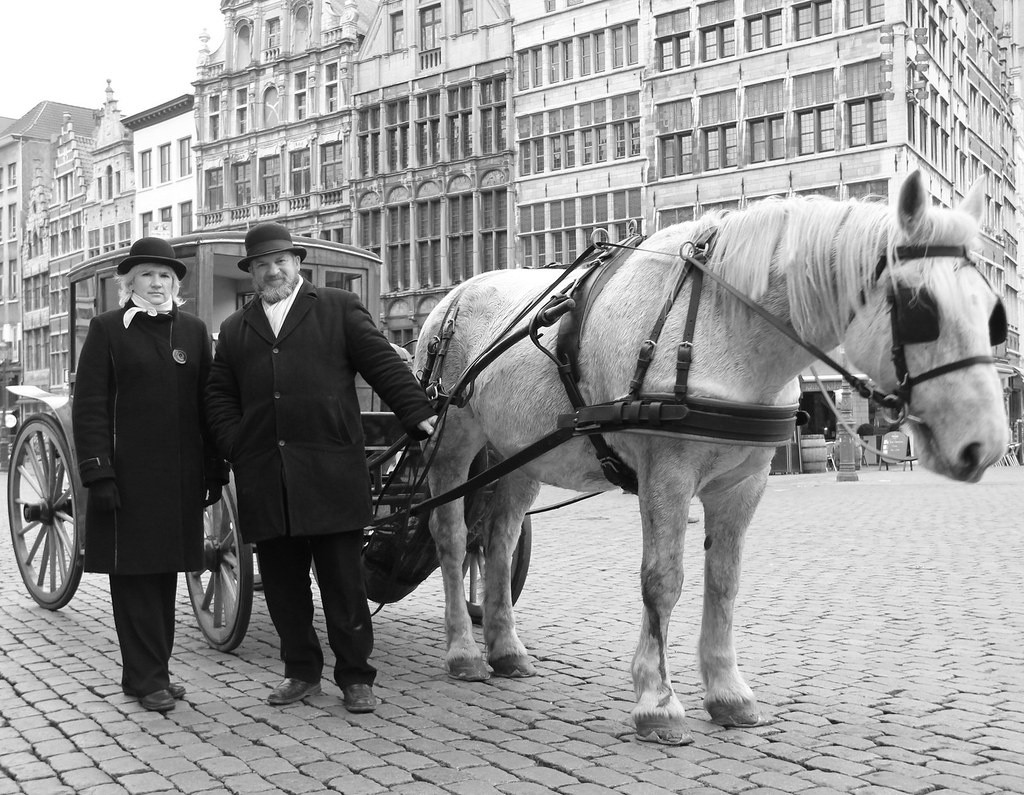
[167,683,185,701]
[141,688,174,712]
[266,678,323,705]
[341,683,377,711]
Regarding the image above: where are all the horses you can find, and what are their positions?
[419,168,1011,741]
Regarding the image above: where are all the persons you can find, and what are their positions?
[203,221,440,712]
[70,236,229,712]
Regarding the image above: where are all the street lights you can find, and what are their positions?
[1,322,13,471]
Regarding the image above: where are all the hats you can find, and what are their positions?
[117,237,186,280]
[237,223,307,274]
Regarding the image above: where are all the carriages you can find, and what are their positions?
[6,171,1008,747]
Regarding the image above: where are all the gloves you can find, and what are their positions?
[205,478,224,508]
[90,478,121,513]
